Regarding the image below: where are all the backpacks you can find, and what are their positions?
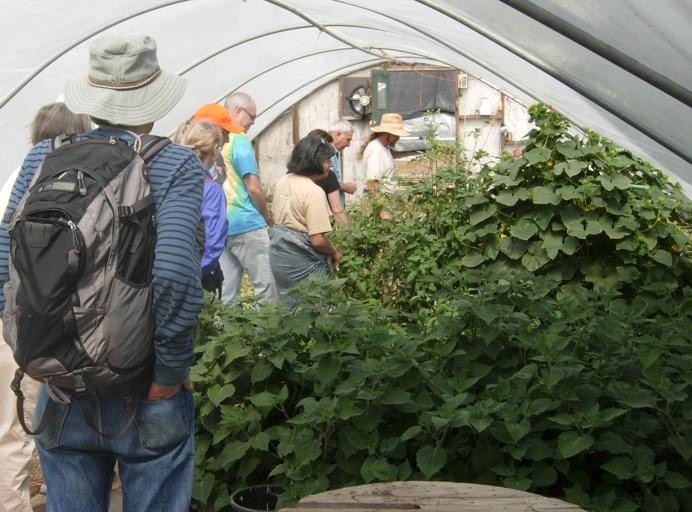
[1,133,172,394]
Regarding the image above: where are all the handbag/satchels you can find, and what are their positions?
[201,261,224,291]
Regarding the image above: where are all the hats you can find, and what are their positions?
[370,113,411,137]
[64,35,188,126]
[190,103,245,134]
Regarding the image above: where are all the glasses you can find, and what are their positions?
[241,108,256,120]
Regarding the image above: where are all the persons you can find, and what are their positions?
[176,92,283,328]
[356,113,406,221]
[1,102,94,511]
[268,120,356,303]
[1,33,205,511]
